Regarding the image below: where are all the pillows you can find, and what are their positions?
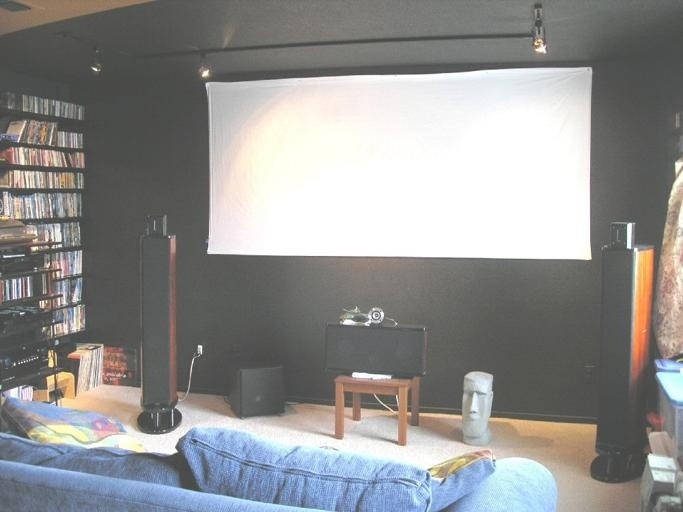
[177,426,433,511]
[0,426,195,493]
[2,394,178,456]
[426,450,496,512]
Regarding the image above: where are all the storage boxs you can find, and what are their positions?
[655,358,683,461]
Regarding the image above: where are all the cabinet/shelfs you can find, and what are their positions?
[0,87,141,388]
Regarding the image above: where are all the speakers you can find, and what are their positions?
[591,244,655,482]
[322,324,429,377]
[136,234,183,434]
[232,366,285,418]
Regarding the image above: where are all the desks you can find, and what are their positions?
[334,373,422,445]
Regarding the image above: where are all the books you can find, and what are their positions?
[0,93,103,401]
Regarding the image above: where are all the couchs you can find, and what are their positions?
[0,457,558,511]
